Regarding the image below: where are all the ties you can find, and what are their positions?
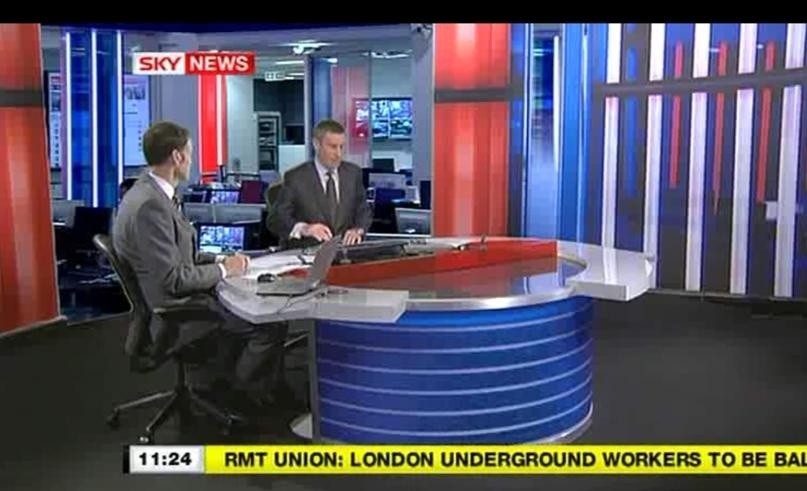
[326,172,338,207]
[174,192,184,219]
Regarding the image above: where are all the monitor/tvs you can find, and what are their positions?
[47,71,152,169]
[207,190,241,203]
[367,173,407,202]
[71,206,114,254]
[394,206,431,235]
[194,222,245,254]
[350,96,414,143]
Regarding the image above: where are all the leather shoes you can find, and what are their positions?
[225,410,291,437]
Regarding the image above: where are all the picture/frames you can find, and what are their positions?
[43,68,64,173]
[122,73,154,170]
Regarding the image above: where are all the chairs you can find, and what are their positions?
[238,180,267,202]
[54,206,115,320]
[92,233,264,445]
[264,181,320,374]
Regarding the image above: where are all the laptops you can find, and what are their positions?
[255,233,344,298]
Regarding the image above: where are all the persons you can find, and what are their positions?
[268,121,373,247]
[111,121,287,435]
